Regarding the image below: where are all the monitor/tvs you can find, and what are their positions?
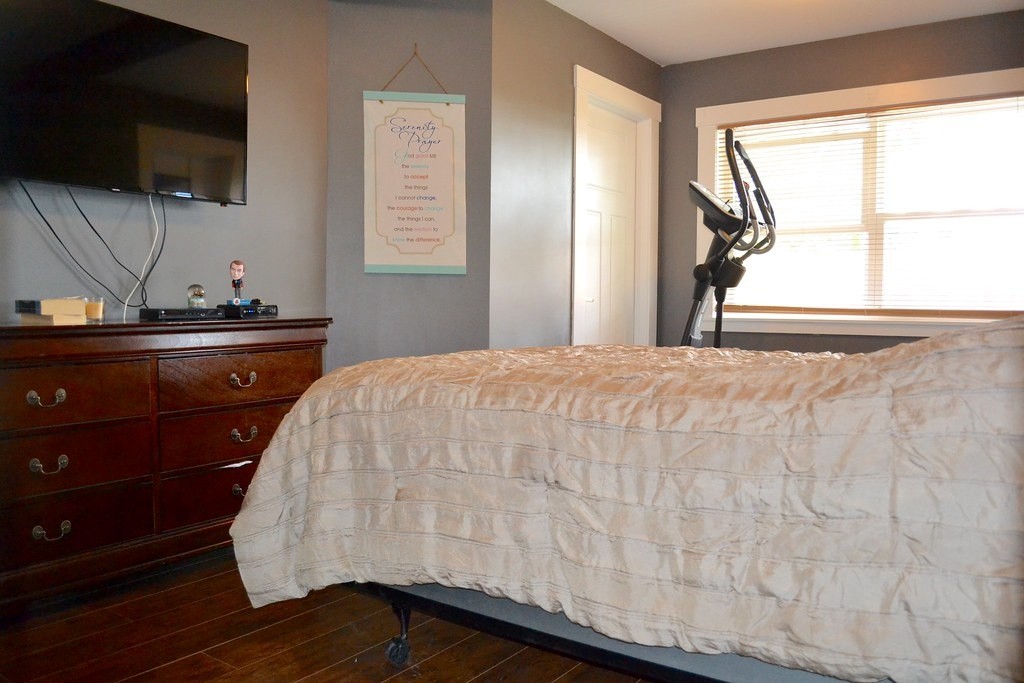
[0,0,249,206]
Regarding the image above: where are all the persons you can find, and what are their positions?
[230,260,246,298]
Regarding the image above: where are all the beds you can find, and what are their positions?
[229,315,1024,683]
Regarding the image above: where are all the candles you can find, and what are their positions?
[85,296,105,319]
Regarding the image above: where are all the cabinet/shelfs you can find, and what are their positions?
[0,317,335,605]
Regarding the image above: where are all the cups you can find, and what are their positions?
[84,297,105,323]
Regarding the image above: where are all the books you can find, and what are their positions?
[15,299,87,325]
